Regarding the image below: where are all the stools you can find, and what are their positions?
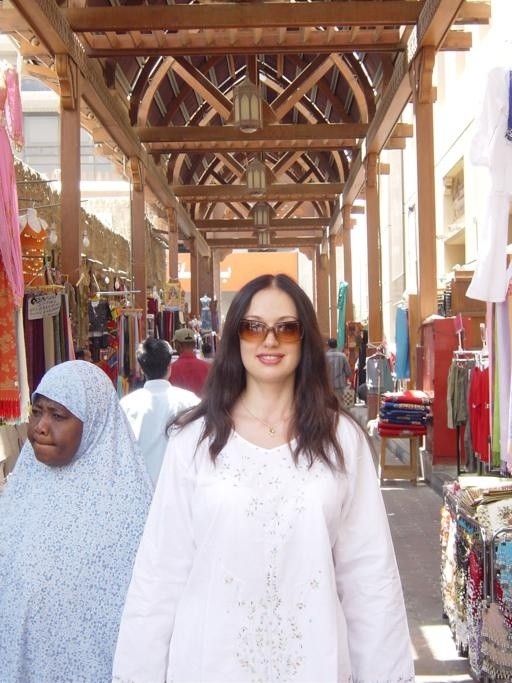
[377,424,426,488]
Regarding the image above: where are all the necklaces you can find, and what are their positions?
[237,391,291,436]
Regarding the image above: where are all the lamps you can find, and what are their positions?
[231,54,275,249]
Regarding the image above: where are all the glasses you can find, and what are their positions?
[236,318,307,346]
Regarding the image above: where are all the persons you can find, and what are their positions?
[213,294,220,328]
[324,338,352,405]
[77,349,94,363]
[199,292,213,329]
[110,272,417,682]
[19,206,50,285]
[0,358,152,682]
[120,337,202,491]
[167,327,215,399]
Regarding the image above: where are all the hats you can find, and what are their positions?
[170,328,195,343]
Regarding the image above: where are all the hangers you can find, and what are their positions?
[365,347,388,362]
[453,349,489,372]
